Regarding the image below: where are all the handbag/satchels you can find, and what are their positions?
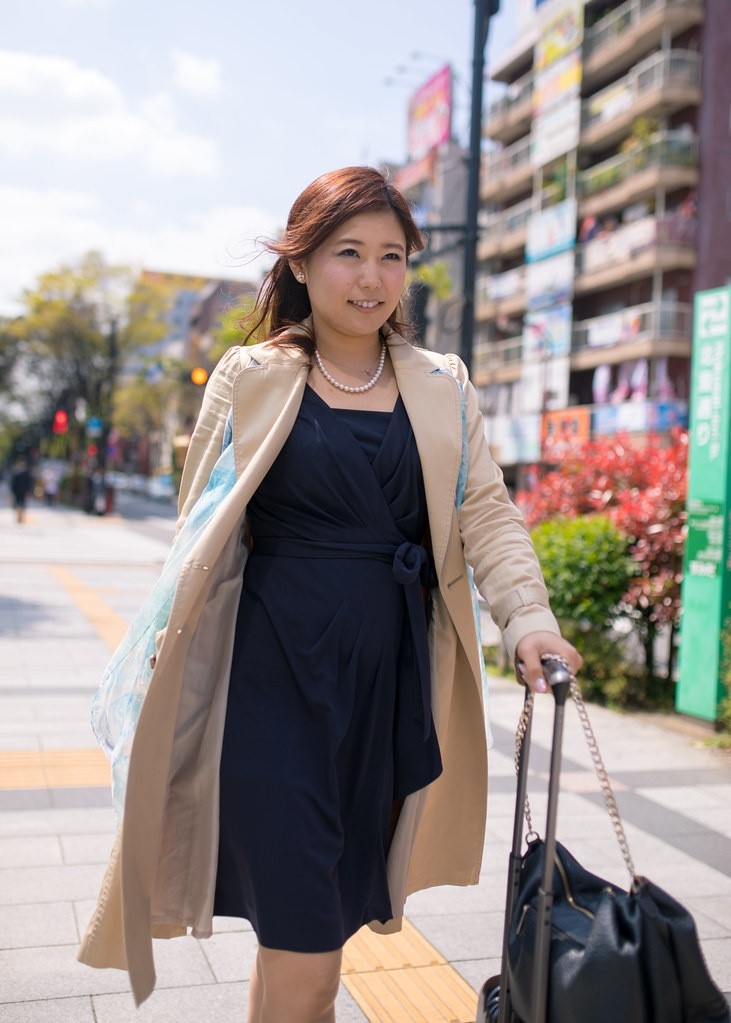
[506,653,730,1023]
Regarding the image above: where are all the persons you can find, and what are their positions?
[77,166,583,1023]
[9,446,113,523]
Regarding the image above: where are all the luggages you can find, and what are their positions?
[476,657,570,1023]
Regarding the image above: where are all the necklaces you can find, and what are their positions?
[315,334,387,393]
